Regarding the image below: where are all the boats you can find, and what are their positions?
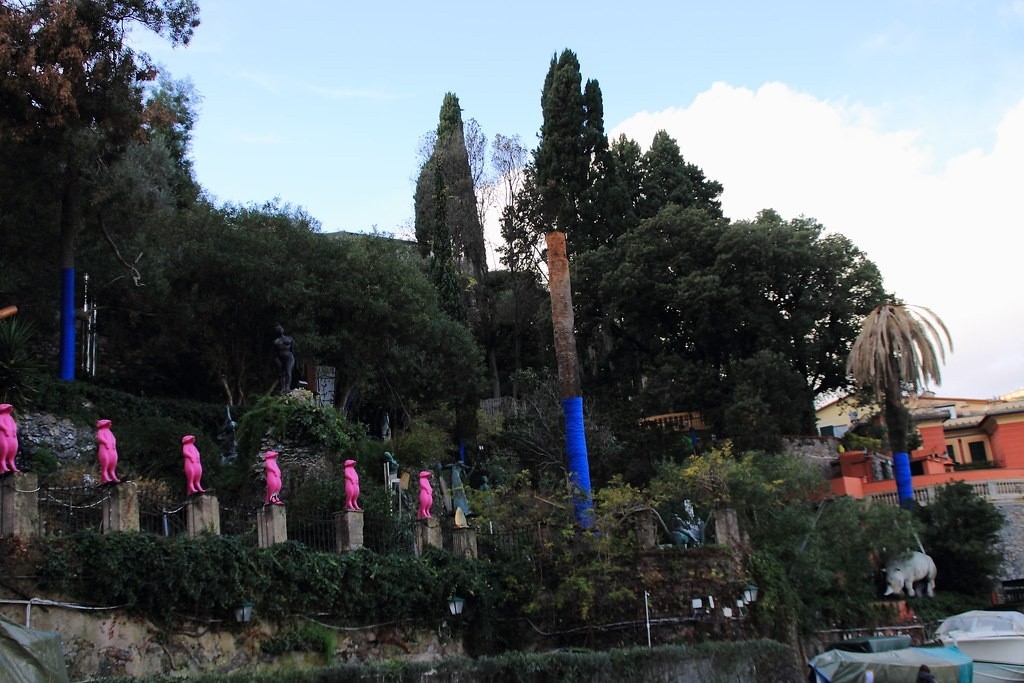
[932,609,1024,666]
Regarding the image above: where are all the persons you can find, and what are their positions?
[272,324,298,395]
[479,475,490,490]
[443,460,474,517]
[916,664,938,683]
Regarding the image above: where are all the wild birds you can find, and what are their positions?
[384,448,397,465]
